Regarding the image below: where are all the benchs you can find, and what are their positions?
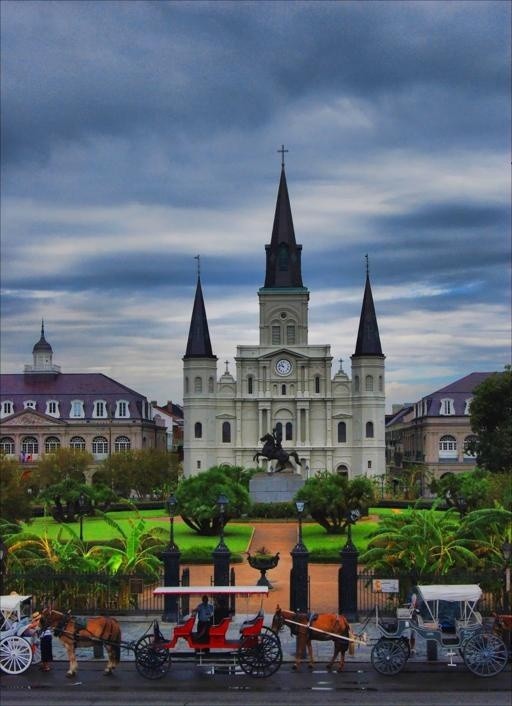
[173,609,265,637]
[455,611,483,630]
[416,612,439,630]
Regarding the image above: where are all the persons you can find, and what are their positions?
[194,596,214,643]
[272,429,282,449]
[40,630,53,671]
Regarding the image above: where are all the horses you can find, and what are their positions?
[253,433,302,473]
[271,603,356,672]
[492,612,512,639]
[36,603,122,679]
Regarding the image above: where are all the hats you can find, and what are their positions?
[9,591,19,596]
[32,612,41,622]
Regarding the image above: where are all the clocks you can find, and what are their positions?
[276,359,292,375]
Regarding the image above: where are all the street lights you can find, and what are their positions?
[338,507,360,623]
[380,475,385,499]
[290,500,311,611]
[161,492,181,623]
[213,493,235,623]
